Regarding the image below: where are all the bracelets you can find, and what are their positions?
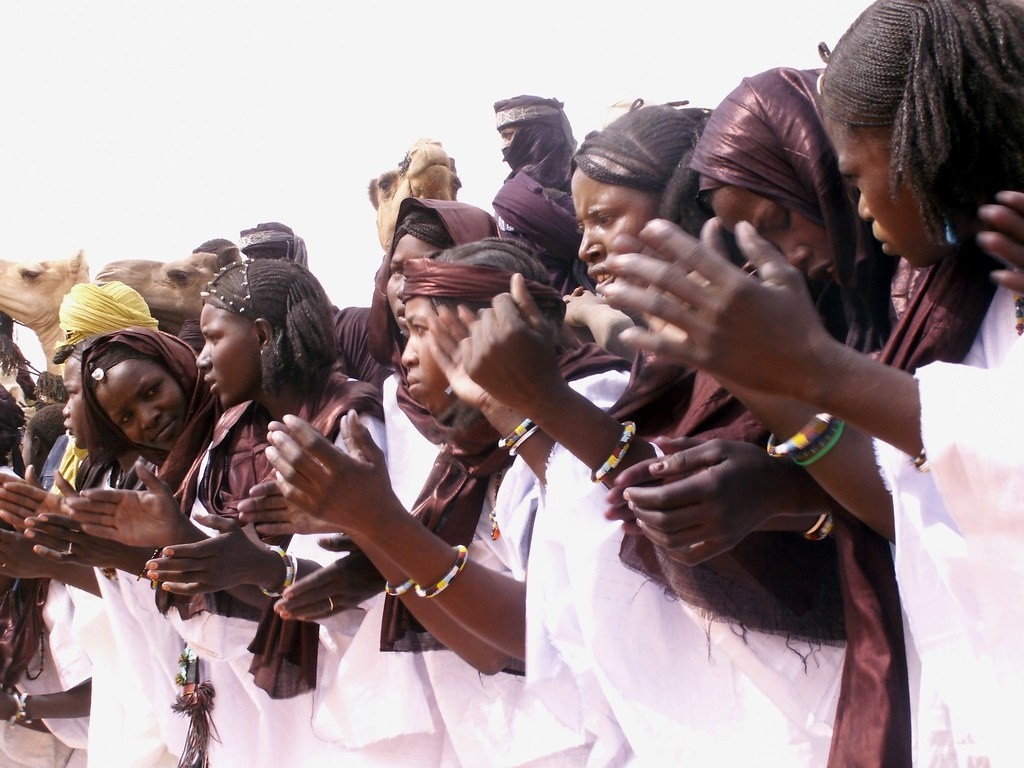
[386,579,414,596]
[102,569,118,580]
[258,546,298,596]
[591,421,635,483]
[137,548,167,590]
[21,695,31,725]
[767,413,844,465]
[415,545,468,598]
[802,513,833,541]
[499,418,538,456]
[908,448,929,473]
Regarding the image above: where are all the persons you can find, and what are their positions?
[193,223,307,272]
[430,102,711,768]
[602,65,918,768]
[490,96,577,235]
[1,329,223,768]
[607,0,1024,768]
[237,196,504,768]
[265,237,634,768]
[560,285,641,359]
[0,280,158,768]
[68,258,387,768]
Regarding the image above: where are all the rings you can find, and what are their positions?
[62,541,73,555]
[329,596,333,611]
[0,563,5,567]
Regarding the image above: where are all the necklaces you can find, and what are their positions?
[490,471,500,540]
[1013,267,1024,336]
[26,633,45,681]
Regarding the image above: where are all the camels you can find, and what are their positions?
[366,137,464,255]
[92,234,244,356]
[0,246,95,387]
